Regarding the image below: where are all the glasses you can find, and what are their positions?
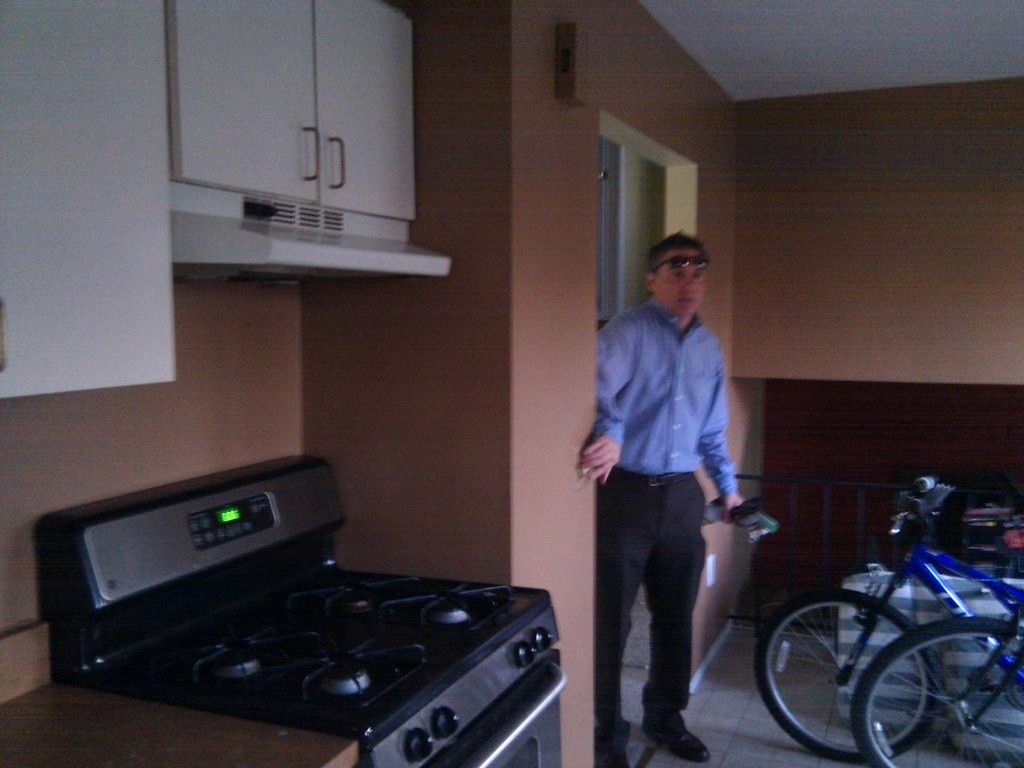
[650,255,710,273]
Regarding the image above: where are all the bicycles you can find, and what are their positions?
[753,470,1024,768]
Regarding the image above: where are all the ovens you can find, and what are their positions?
[422,648,568,768]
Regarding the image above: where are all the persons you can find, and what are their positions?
[581,234,742,768]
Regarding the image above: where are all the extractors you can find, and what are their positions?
[170,180,452,284]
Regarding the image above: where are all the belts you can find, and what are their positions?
[612,471,693,486]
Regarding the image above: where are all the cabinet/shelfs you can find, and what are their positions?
[0,1,179,399]
[166,0,418,221]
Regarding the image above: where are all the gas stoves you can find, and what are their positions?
[34,455,559,768]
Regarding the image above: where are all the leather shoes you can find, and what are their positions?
[640,713,711,763]
[594,728,629,768]
